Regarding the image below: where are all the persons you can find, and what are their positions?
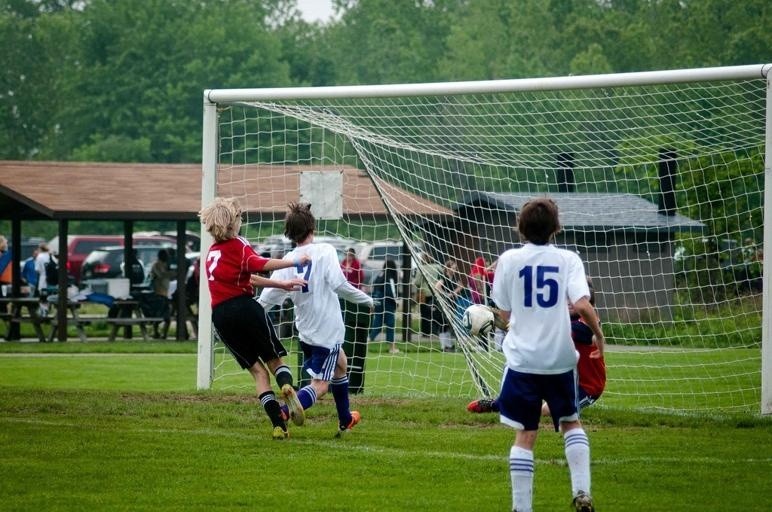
[465,278,606,414]
[257,201,375,431]
[251,234,491,353]
[1,235,76,316]
[492,197,606,512]
[198,194,310,438]
[118,248,200,340]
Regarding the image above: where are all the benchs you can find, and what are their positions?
[0,296,198,342]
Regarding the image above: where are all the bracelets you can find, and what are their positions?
[595,332,603,341]
[293,257,300,267]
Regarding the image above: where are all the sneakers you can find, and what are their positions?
[338,411,361,433]
[573,494,595,511]
[468,398,495,413]
[272,426,290,441]
[363,335,496,357]
[281,383,307,427]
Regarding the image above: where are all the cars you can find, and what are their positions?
[361,239,424,280]
[674,236,762,283]
[156,232,201,252]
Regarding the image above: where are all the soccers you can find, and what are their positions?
[461,303,493,336]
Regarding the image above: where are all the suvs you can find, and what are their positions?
[81,245,188,286]
[39,232,186,281]
